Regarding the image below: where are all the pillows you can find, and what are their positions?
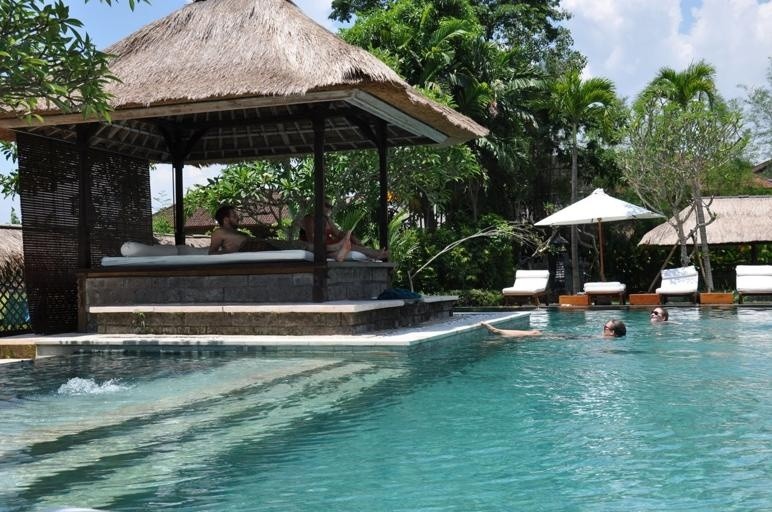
[102,239,126,258]
[122,242,178,258]
[176,245,213,256]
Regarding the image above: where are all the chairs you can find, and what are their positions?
[501,266,557,308]
[734,261,771,304]
[582,280,629,305]
[655,265,700,305]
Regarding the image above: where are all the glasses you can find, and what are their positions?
[326,202,334,209]
[651,311,658,315]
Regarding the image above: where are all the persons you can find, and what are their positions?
[478,318,630,339]
[300,197,391,259]
[207,206,352,265]
[648,305,669,322]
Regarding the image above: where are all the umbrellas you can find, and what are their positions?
[533,188,666,282]
